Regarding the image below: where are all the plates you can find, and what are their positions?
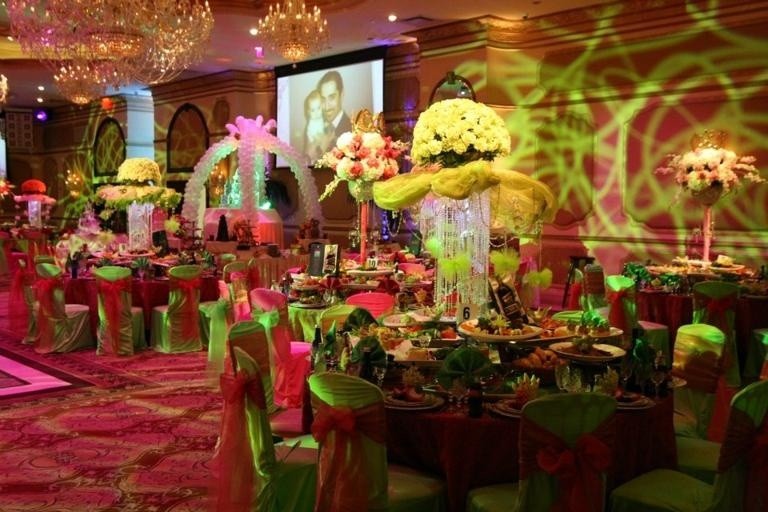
[341,280,380,290]
[393,347,445,368]
[381,311,457,329]
[489,399,521,419]
[613,393,656,410]
[456,319,542,341]
[382,391,443,410]
[548,342,626,364]
[289,302,325,308]
[347,270,394,275]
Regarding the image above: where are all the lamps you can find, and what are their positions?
[1,1,216,105]
[249,1,330,70]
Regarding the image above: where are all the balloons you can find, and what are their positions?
[178,114,325,242]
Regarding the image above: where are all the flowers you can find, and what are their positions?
[414,95,512,166]
[651,128,765,235]
[20,179,47,195]
[313,126,412,207]
[91,156,183,211]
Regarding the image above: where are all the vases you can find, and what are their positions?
[347,180,376,267]
[28,200,42,228]
[430,188,493,320]
[687,180,727,268]
[126,200,155,251]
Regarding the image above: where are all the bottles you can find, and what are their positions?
[310,326,337,375]
[70,254,78,279]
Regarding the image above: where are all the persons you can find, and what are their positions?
[303,71,353,162]
[303,91,325,144]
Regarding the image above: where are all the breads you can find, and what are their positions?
[510,347,561,368]
